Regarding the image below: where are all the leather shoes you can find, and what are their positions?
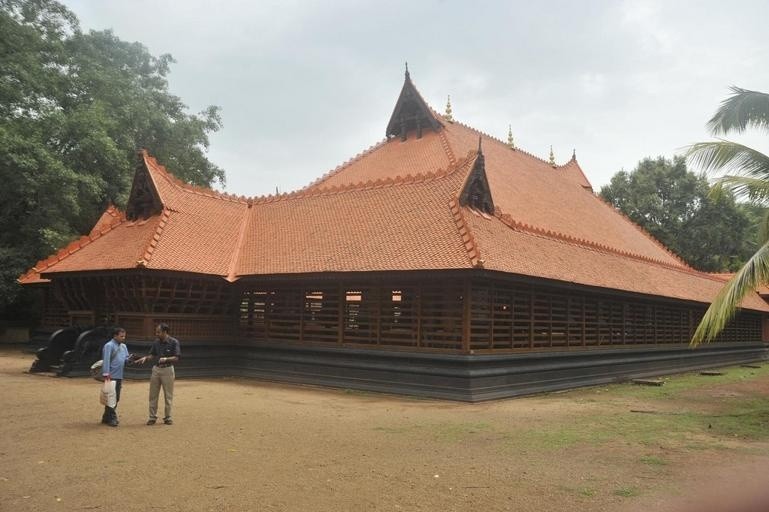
[156,363,172,368]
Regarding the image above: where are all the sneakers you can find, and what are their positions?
[147,416,158,425]
[164,417,172,424]
[102,414,119,426]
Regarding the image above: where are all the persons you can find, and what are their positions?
[102,328,134,426]
[134,323,180,425]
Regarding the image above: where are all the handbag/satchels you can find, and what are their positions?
[101,378,116,408]
[91,360,104,381]
